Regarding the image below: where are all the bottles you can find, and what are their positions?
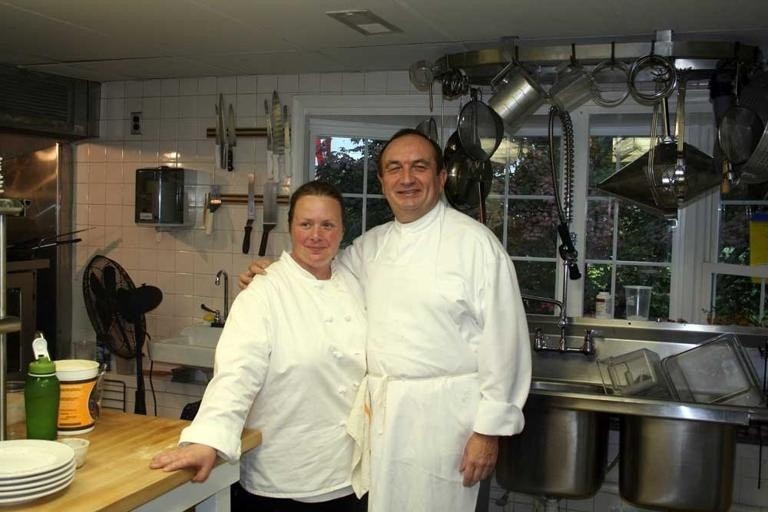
[596,291,613,322]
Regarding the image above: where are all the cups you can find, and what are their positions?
[624,285,653,320]
[22,334,60,440]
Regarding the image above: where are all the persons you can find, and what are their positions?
[238,128,534,511]
[151,179,371,511]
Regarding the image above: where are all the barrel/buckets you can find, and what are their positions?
[487,71,547,129]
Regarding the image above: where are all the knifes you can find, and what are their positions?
[215,93,236,171]
[242,91,293,256]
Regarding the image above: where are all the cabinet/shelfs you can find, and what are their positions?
[202,127,289,231]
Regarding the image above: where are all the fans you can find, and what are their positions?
[83,255,163,415]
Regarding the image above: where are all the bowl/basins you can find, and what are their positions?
[58,437,90,467]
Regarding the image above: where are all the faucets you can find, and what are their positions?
[214,270,229,322]
[522,294,565,349]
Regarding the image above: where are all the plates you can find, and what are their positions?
[0,440,77,506]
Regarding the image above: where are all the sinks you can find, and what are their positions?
[147,325,225,369]
[617,387,739,512]
[500,375,615,497]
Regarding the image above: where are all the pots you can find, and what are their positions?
[489,60,547,136]
[444,88,503,212]
